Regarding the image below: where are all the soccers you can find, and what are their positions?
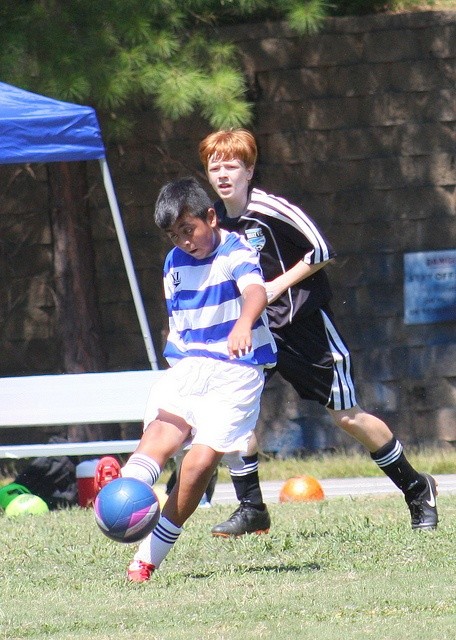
[280,475,324,504]
[1,484,31,511]
[93,477,160,543]
[5,494,49,521]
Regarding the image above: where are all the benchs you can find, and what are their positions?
[1,369,207,506]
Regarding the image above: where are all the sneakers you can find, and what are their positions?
[94,457,122,502]
[404,473,438,531]
[128,560,156,583]
[211,503,270,538]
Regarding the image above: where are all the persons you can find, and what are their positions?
[200,127,439,539]
[94,175,278,583]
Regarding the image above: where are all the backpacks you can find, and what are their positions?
[15,435,79,510]
